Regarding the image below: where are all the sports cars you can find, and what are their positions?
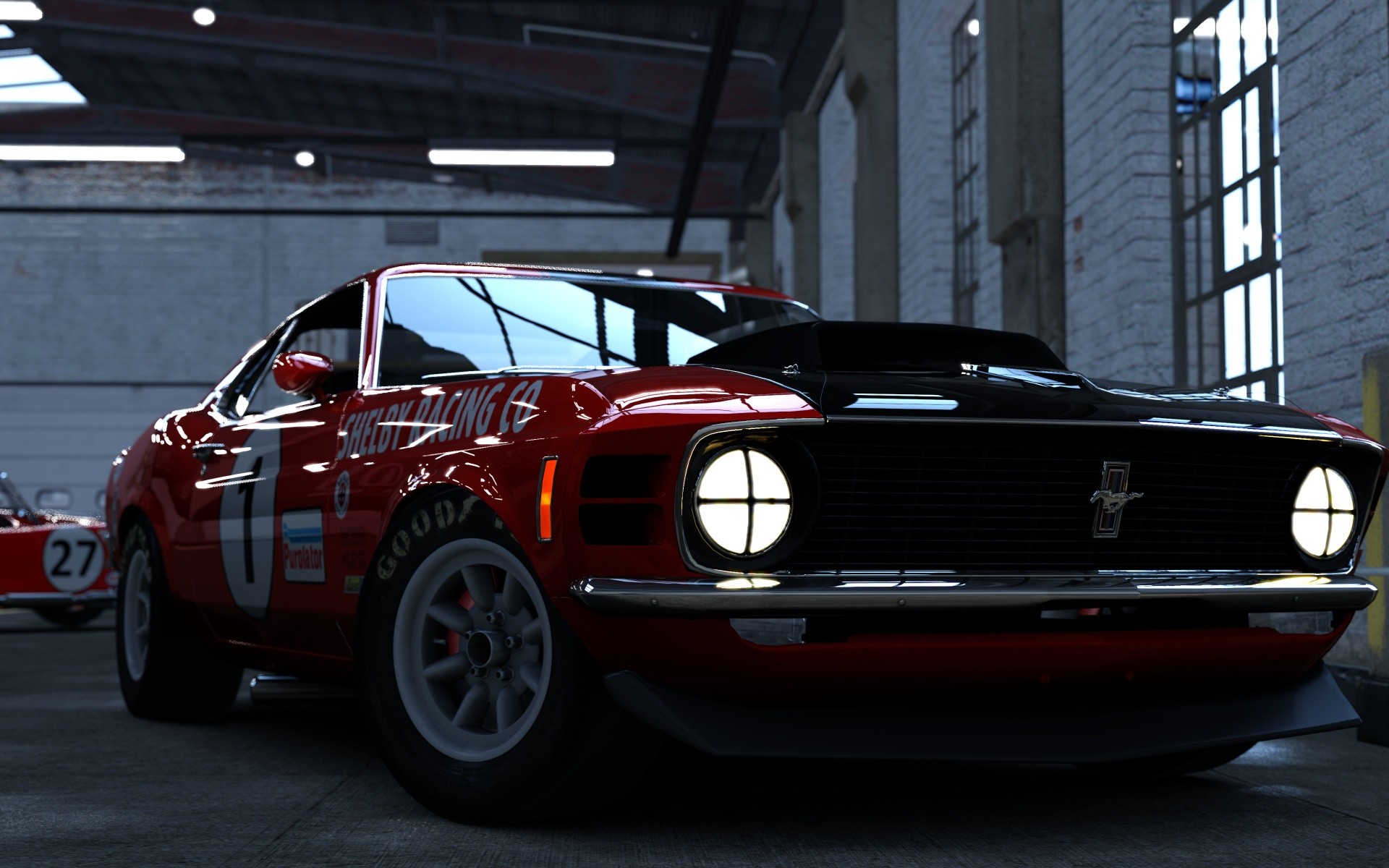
[104,262,1388,831]
[0,470,120,631]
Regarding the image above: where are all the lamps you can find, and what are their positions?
[426,138,615,167]
[0,132,187,162]
